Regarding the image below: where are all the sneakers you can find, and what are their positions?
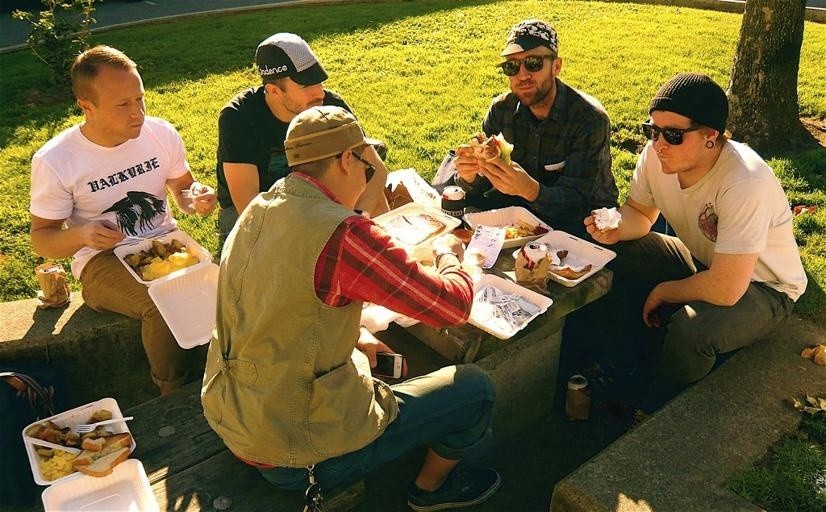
[407,468,501,511]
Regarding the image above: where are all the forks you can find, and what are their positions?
[77,415,133,434]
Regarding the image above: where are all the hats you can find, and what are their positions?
[650,72,728,133]
[255,32,328,86]
[284,105,382,167]
[500,19,558,56]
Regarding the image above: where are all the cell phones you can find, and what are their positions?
[369,352,403,378]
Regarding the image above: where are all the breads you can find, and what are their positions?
[556,264,594,279]
[73,433,131,477]
[592,207,622,237]
[383,215,447,243]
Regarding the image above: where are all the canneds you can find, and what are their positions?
[38,266,69,308]
[524,241,548,271]
[567,374,589,397]
[442,186,465,229]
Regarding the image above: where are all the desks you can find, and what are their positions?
[388,248,608,439]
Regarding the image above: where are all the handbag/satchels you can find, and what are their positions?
[1,370,56,506]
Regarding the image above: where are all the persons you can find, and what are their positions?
[201,105,502,512]
[453,19,620,270]
[28,43,218,395]
[216,32,390,239]
[572,73,808,433]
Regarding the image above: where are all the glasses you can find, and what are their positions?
[642,120,705,145]
[499,55,554,75]
[336,150,376,183]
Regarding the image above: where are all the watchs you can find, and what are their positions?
[353,209,372,220]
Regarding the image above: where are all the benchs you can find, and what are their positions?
[126,366,226,483]
[151,446,367,512]
[1,281,142,389]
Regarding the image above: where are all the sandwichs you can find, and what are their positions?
[466,131,511,178]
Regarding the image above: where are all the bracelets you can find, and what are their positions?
[435,251,461,269]
